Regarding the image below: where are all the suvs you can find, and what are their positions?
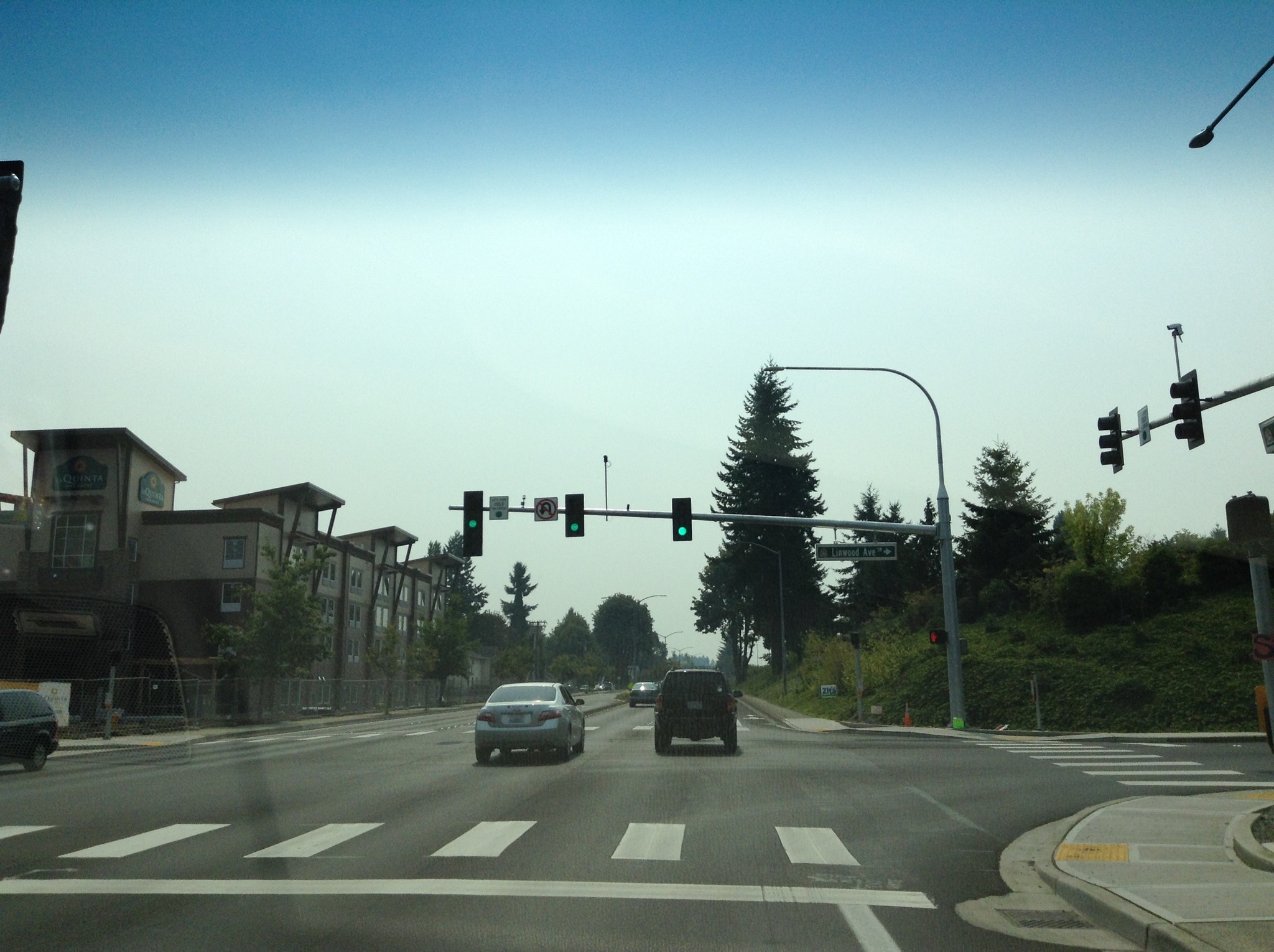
[656,667,743,751]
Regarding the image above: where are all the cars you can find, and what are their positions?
[476,682,587,761]
[630,681,659,706]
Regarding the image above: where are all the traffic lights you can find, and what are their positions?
[563,493,586,537]
[927,627,948,645]
[462,490,484,557]
[1097,406,1126,474]
[1171,368,1207,451]
[671,497,694,541]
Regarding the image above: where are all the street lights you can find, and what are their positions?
[764,363,972,730]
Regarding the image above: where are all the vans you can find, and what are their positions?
[0,689,58,773]
